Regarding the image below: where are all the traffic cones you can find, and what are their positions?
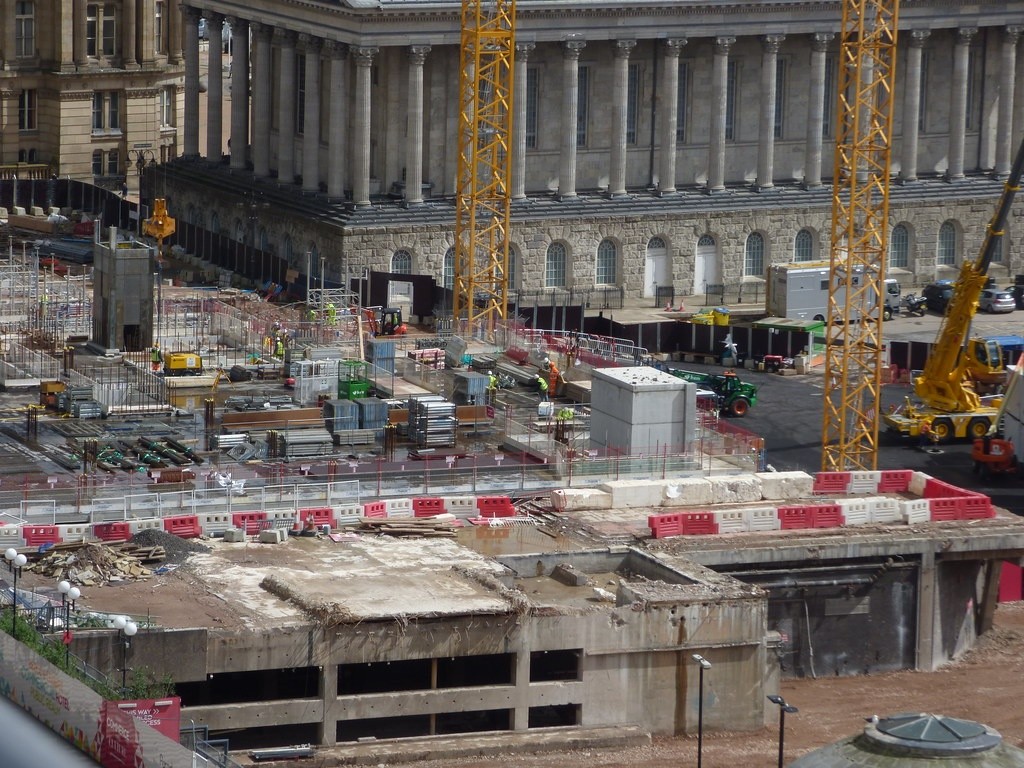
[664,301,672,312]
[679,299,687,311]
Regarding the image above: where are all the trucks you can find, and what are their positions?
[764,257,901,326]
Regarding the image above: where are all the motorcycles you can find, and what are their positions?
[902,290,930,317]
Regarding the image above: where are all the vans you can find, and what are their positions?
[921,278,958,315]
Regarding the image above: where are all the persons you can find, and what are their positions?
[557,406,573,420]
[487,370,500,403]
[41,289,49,317]
[328,304,336,326]
[152,343,163,371]
[543,358,551,370]
[549,362,563,397]
[393,315,397,325]
[272,337,283,361]
[534,373,548,402]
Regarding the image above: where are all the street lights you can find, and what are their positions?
[57,580,81,669]
[687,651,714,768]
[112,616,138,701]
[124,147,158,238]
[3,547,28,637]
[765,692,798,767]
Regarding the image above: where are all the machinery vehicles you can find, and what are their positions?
[348,303,407,336]
[666,364,766,417]
[883,135,1024,448]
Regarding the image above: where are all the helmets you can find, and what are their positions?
[487,371,492,374]
[275,337,280,341]
[154,343,159,347]
[564,407,568,412]
[534,375,539,379]
[544,358,548,363]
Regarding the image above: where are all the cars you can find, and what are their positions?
[977,285,1024,314]
